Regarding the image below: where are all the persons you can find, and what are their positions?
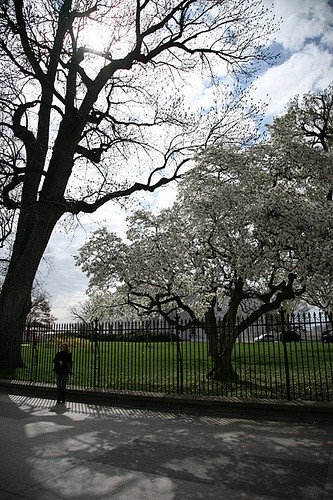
[53,342,74,404]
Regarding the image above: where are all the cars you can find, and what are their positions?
[278,331,302,342]
[321,329,333,343]
[254,333,275,342]
